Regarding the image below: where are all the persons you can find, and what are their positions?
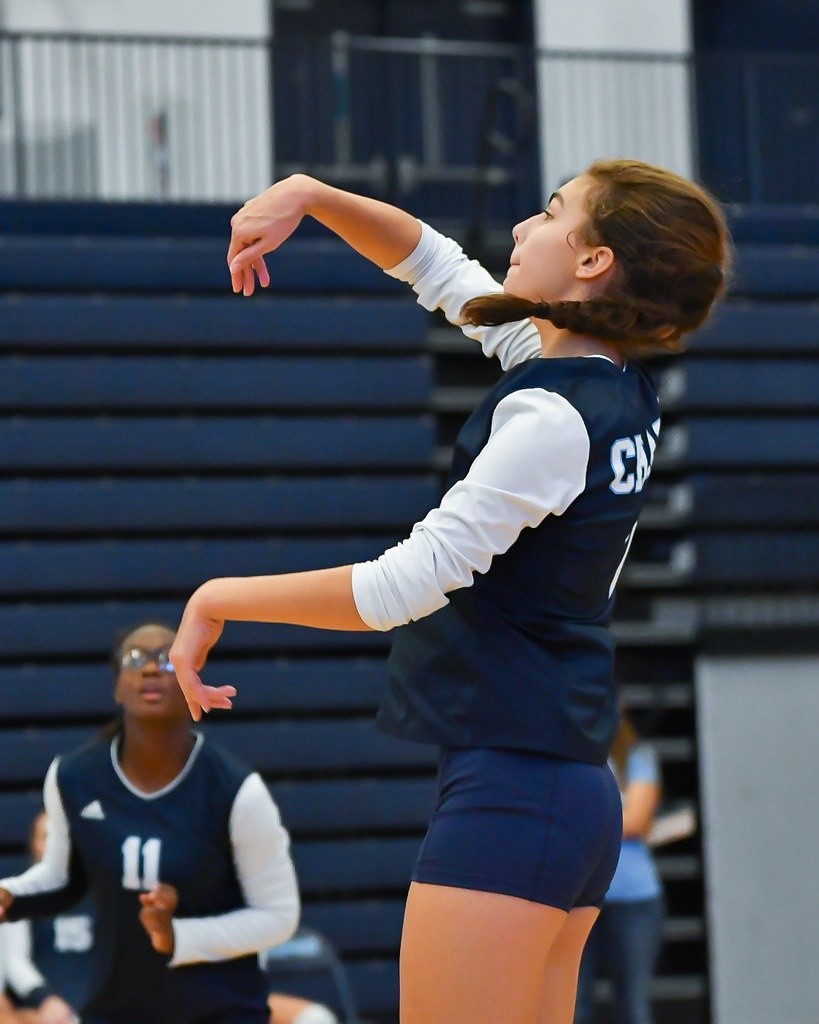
[0,624,302,1024]
[165,160,729,1024]
[0,810,338,1024]
[573,700,663,1024]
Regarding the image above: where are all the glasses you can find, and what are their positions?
[118,645,174,673]
